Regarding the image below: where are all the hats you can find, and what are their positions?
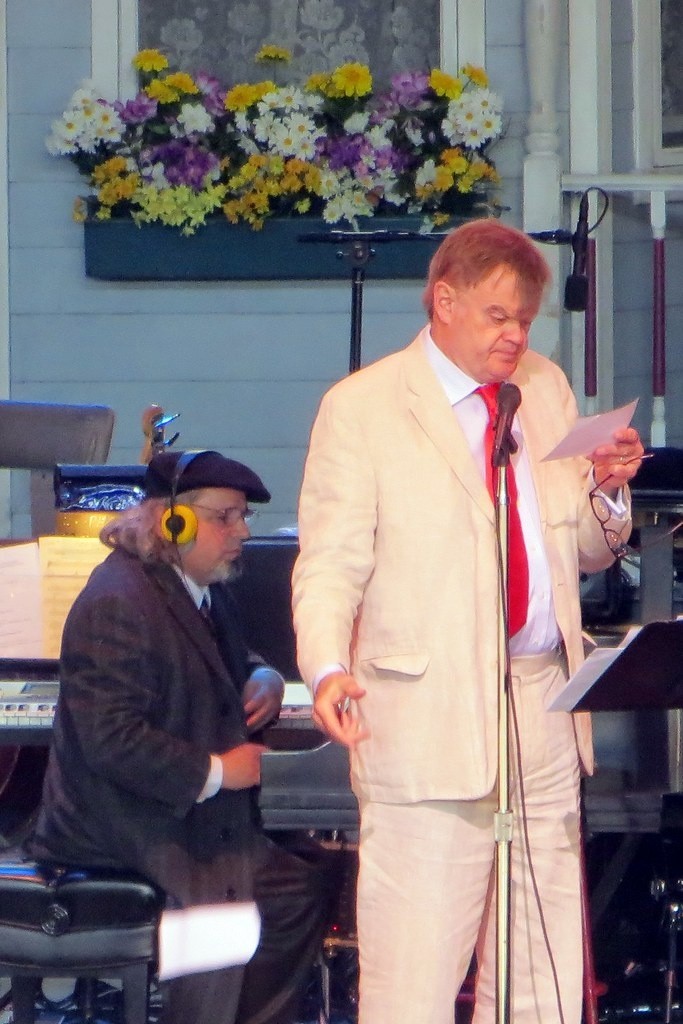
[142,451,272,506]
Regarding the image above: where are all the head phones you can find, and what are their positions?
[161,450,216,544]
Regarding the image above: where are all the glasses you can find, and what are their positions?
[186,503,262,528]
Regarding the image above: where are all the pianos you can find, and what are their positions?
[0,681,327,752]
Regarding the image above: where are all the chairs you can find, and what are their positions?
[1,399,115,549]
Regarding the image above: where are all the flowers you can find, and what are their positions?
[43,44,514,227]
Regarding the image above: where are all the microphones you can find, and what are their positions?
[490,383,522,469]
[564,195,592,313]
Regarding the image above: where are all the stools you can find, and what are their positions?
[0,848,162,1024]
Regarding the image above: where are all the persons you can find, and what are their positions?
[292,216,645,1024]
[35,451,337,1024]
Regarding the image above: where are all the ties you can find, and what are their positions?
[199,598,216,637]
[474,380,531,641]
